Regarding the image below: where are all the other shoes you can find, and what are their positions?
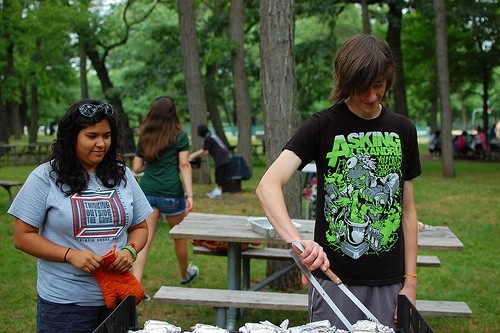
[206,189,222,198]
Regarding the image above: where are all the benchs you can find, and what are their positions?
[153,285,473,328]
[0,180,23,202]
[192,245,442,318]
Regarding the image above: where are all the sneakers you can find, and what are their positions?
[143,292,151,302]
[180,263,199,287]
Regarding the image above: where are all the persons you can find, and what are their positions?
[255,33,422,333]
[189,124,233,199]
[7,99,153,333]
[130,95,198,300]
[429,122,500,163]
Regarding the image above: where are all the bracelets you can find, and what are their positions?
[121,243,139,262]
[185,192,193,199]
[404,273,418,281]
[63,247,73,262]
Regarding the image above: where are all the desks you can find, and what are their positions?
[0,141,52,165]
[169,211,466,332]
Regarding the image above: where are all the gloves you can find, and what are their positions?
[89,243,145,312]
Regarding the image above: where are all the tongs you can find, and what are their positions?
[288,240,381,333]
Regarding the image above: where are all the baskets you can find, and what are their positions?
[247,217,302,238]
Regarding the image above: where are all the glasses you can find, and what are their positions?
[77,102,117,117]
